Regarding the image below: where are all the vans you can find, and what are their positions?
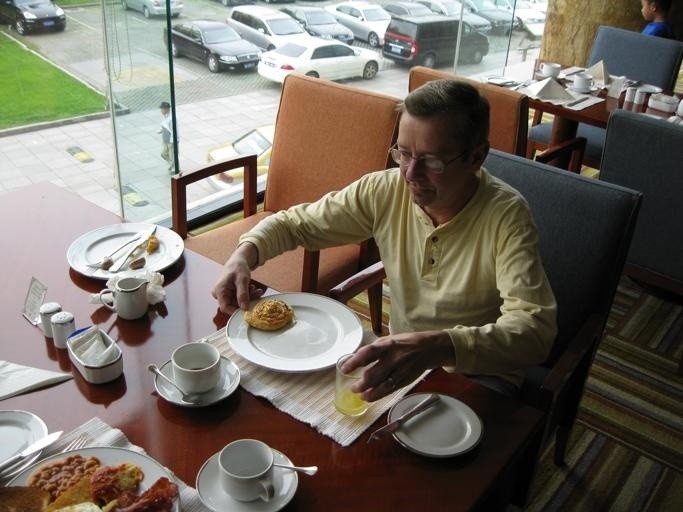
[380,15,492,69]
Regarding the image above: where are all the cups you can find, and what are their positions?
[334,353,373,417]
[624,87,646,105]
[99,277,149,321]
[218,439,274,503]
[538,62,561,77]
[170,338,220,393]
[574,73,594,90]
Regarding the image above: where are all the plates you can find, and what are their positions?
[487,77,521,86]
[636,79,663,94]
[386,392,484,459]
[0,447,183,512]
[536,71,566,81]
[225,291,363,374]
[195,448,298,512]
[153,355,241,409]
[66,223,184,281]
[566,83,592,93]
[0,410,48,481]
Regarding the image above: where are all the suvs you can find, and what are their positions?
[227,4,312,53]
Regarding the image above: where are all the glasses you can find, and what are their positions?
[389,142,473,174]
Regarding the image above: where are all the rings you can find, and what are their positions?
[386,376,396,390]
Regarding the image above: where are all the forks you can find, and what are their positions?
[86,230,144,269]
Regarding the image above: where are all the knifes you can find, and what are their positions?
[0,431,63,471]
[366,393,440,445]
[566,69,585,77]
[109,225,157,274]
[567,97,589,106]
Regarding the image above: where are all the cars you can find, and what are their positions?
[324,1,391,48]
[381,1,431,15]
[1,1,68,34]
[164,20,259,72]
[123,0,182,18]
[280,5,354,45]
[203,120,275,189]
[420,0,546,37]
[257,35,381,82]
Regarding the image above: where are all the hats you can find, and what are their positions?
[160,102,170,109]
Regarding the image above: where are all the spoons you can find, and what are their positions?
[146,364,207,404]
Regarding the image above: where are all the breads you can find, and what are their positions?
[0,477,103,512]
[244,298,294,332]
[146,235,159,252]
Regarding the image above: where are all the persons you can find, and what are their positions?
[639,0,672,37]
[214,80,559,404]
[158,102,180,175]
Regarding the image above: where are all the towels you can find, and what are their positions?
[0,361,75,401]
[581,59,613,87]
[200,310,444,448]
[0,418,216,511]
[526,76,576,102]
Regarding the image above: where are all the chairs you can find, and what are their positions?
[598,108,683,309]
[407,65,588,175]
[169,72,407,300]
[527,24,683,170]
[328,149,645,466]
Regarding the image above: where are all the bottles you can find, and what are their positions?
[51,312,75,349]
[623,101,643,114]
[39,302,62,338]
